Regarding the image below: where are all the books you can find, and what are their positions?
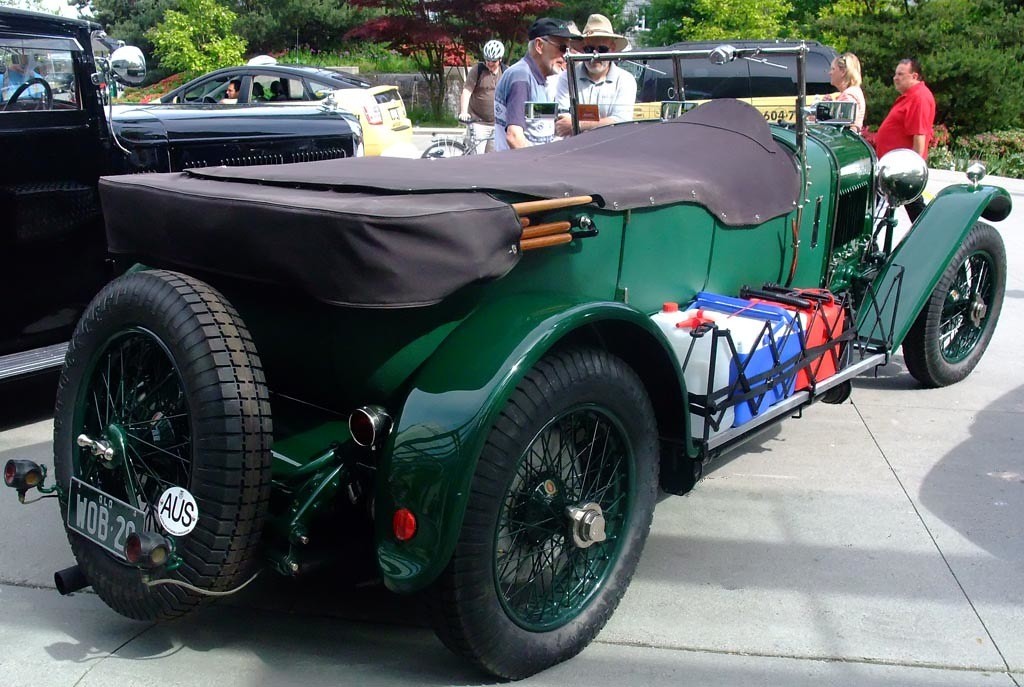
[524,102,559,137]
[577,103,600,122]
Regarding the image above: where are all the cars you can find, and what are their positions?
[143,54,414,157]
[4,36,1013,684]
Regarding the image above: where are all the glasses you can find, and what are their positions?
[541,36,567,52]
[583,40,615,54]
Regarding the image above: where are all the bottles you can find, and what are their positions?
[650,302,735,438]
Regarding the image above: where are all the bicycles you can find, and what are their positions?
[419,114,558,160]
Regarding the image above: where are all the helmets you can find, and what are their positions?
[483,39,505,61]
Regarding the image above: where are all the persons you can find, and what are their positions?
[806,52,865,134]
[553,14,637,139]
[217,79,241,103]
[0,53,47,101]
[860,57,935,223]
[546,21,582,103]
[492,17,585,153]
[458,39,511,155]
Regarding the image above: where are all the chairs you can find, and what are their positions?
[269,81,287,101]
[252,82,268,102]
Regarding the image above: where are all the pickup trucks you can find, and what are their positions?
[1,6,367,386]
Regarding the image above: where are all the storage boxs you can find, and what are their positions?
[685,292,807,427]
[750,291,851,390]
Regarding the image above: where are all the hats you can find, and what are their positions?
[569,13,629,52]
[526,16,583,40]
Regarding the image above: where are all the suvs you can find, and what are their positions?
[619,39,841,124]
